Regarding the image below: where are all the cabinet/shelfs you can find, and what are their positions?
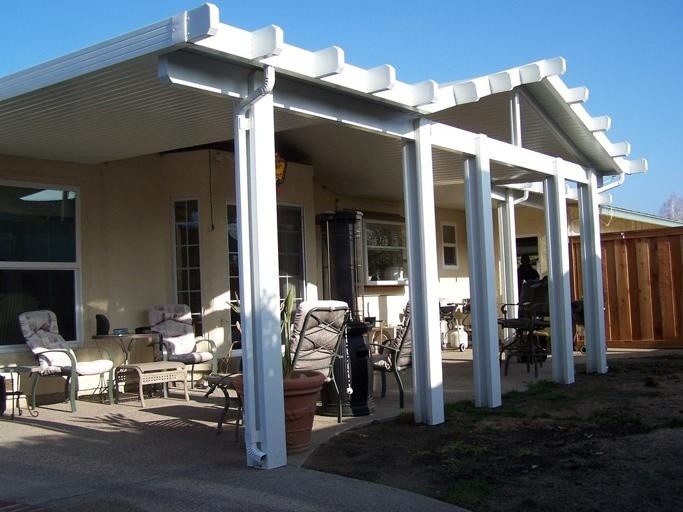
[359,217,409,285]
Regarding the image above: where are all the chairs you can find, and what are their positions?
[499,278,549,377]
[20,312,112,414]
[209,300,343,442]
[370,298,414,409]
[148,305,217,394]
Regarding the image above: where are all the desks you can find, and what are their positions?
[91,332,160,399]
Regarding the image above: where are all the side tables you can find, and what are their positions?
[1,366,61,418]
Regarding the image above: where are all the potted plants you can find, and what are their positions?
[224,285,324,454]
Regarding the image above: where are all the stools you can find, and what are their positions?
[117,362,187,409]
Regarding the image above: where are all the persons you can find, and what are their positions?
[517,255,540,302]
